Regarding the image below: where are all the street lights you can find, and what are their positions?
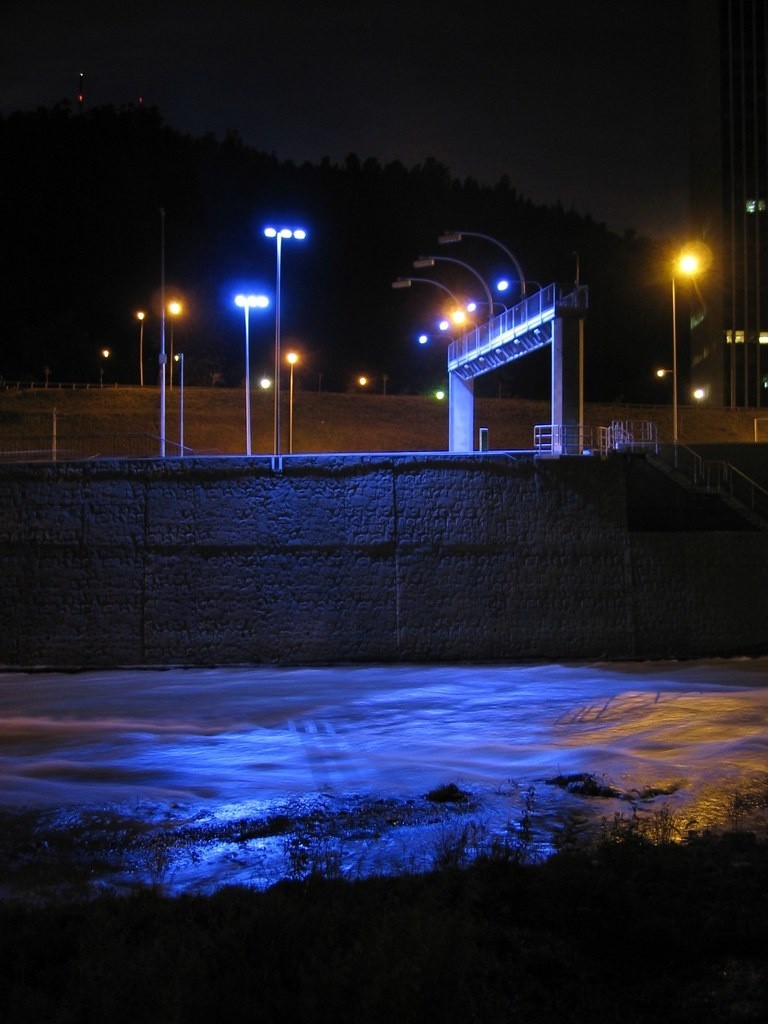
[265,224,307,454]
[99,350,109,389]
[671,255,699,444]
[438,230,527,326]
[413,255,494,323]
[234,294,270,451]
[137,311,145,387]
[286,354,298,454]
[168,303,183,391]
[392,277,467,356]
